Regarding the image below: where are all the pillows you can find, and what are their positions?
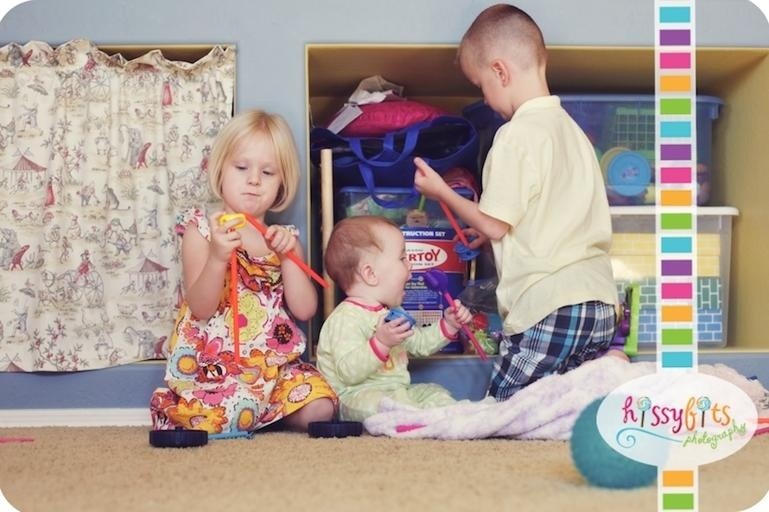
[325,102,448,136]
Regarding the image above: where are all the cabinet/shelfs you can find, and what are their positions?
[0,38,237,364]
[306,43,769,436]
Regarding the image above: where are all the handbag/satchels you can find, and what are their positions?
[309,115,477,208]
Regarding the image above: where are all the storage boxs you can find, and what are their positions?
[606,206,739,349]
[337,186,475,355]
[556,93,724,206]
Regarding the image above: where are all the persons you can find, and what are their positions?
[316,217,473,427]
[414,4,618,402]
[150,111,339,438]
